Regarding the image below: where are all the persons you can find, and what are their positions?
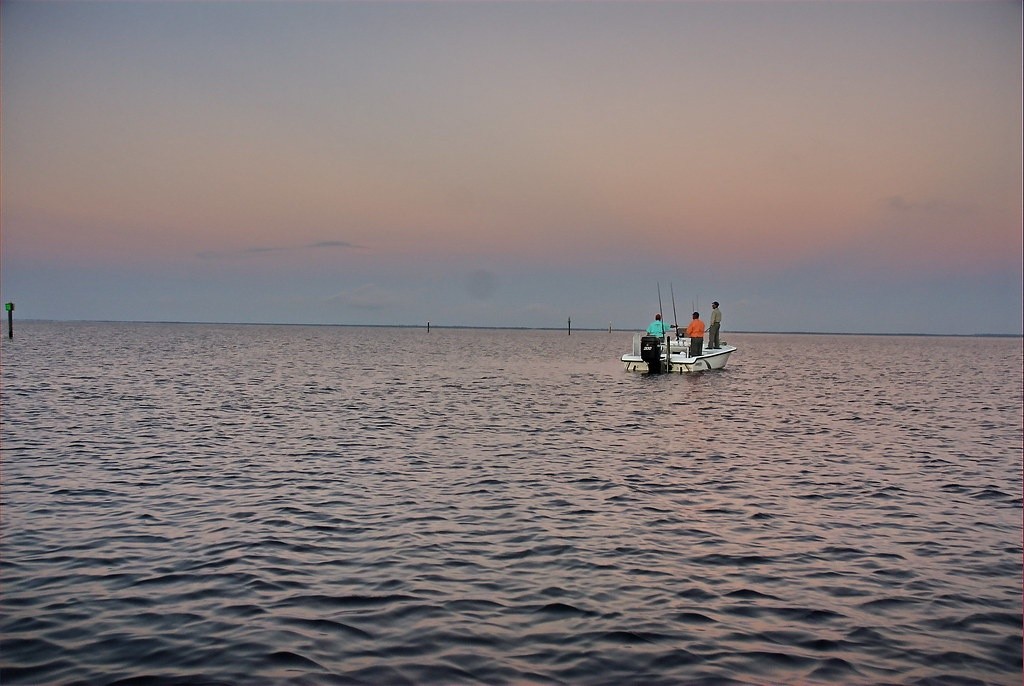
[646,314,677,341]
[687,312,704,356]
[705,302,721,348]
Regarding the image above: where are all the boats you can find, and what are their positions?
[621,280,735,374]
